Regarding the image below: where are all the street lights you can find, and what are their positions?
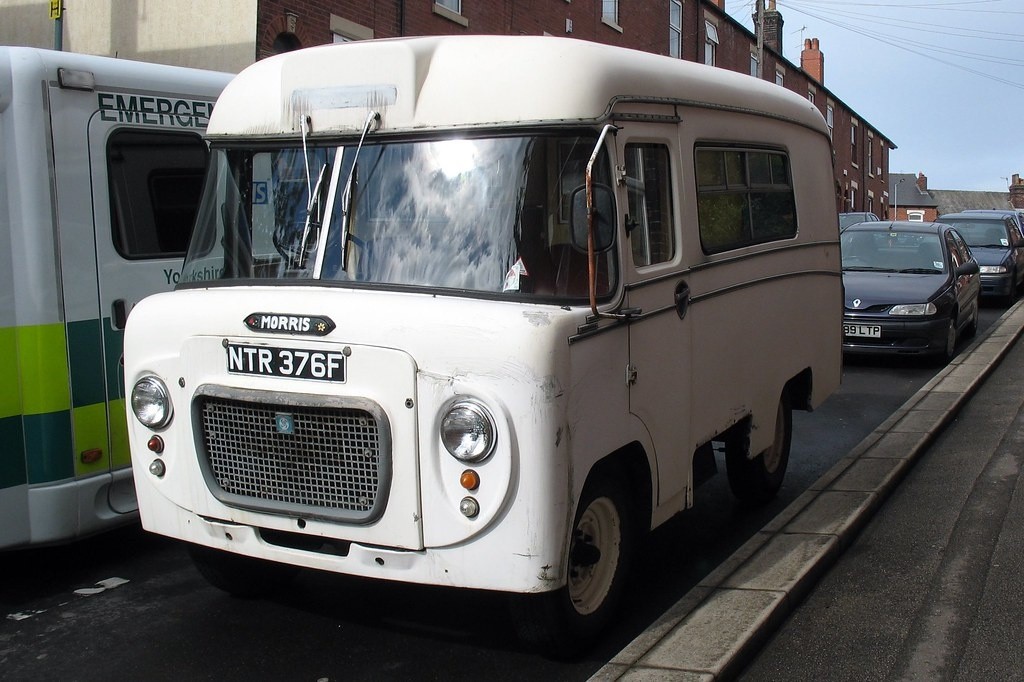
[894,178,905,220]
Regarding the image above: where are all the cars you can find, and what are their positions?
[936,212,1024,302]
[839,212,880,240]
[837,222,983,363]
[951,209,1023,250]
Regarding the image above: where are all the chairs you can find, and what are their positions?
[916,243,942,269]
[984,229,1004,245]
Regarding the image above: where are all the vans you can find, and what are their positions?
[1,50,244,555]
[120,33,849,661]
